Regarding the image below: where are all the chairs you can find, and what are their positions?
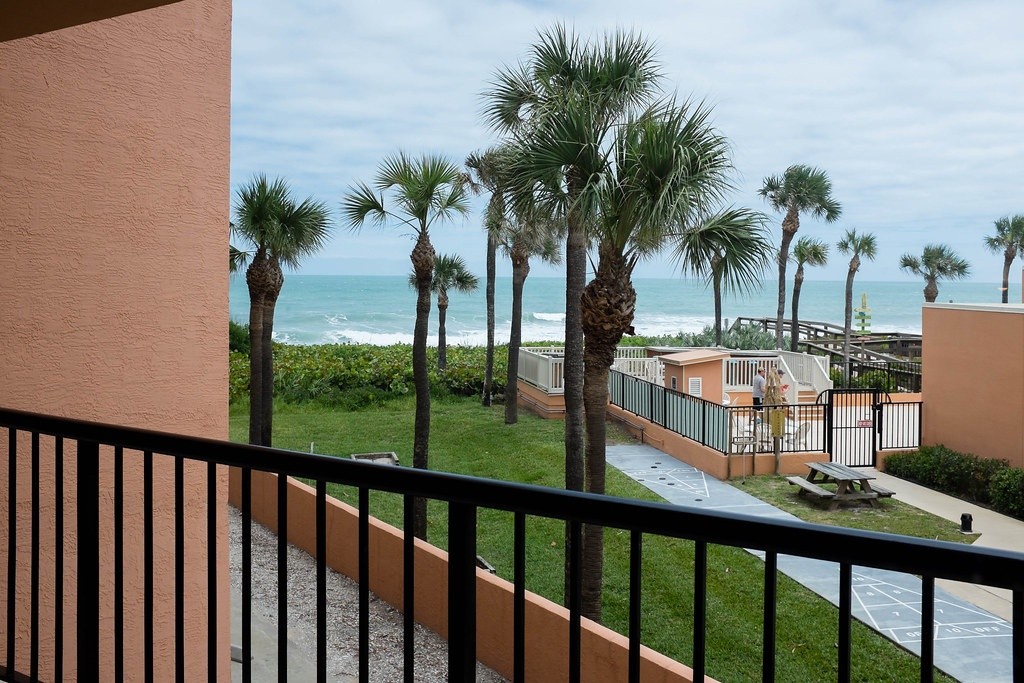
[723,393,811,450]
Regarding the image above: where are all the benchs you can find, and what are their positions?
[853,479,897,498]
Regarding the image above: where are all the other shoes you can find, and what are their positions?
[751,415,761,421]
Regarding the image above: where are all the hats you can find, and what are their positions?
[776,369,785,374]
[759,367,766,372]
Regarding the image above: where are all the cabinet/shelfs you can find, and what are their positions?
[785,475,836,498]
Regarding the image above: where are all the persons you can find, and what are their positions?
[753,367,786,423]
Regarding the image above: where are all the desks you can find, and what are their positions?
[798,461,879,511]
[723,399,730,405]
